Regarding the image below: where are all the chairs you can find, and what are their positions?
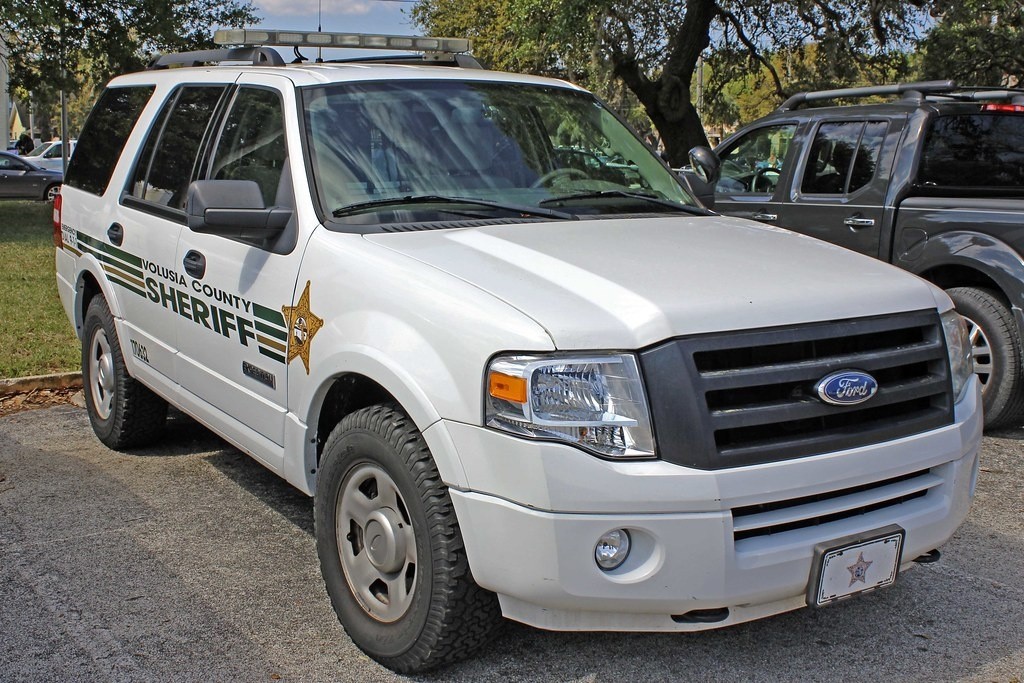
[232,114,352,208]
[425,124,513,190]
[817,140,871,193]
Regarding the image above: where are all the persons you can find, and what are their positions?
[15,134,34,155]
[39,125,61,144]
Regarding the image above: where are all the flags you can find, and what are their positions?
[8,101,23,139]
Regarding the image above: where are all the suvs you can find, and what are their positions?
[50,29,985,677]
[675,79,1024,430]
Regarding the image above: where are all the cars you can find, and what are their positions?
[552,141,650,190]
[19,139,78,171]
[0,150,63,200]
